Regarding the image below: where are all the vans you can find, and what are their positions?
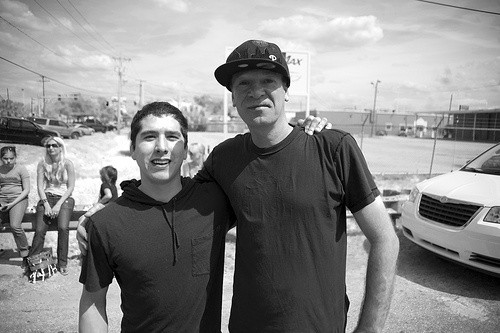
[33,117,83,140]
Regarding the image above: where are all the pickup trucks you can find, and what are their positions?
[0,115,60,148]
[79,119,108,134]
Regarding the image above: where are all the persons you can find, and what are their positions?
[78,163,117,266]
[30,127,75,275]
[80,100,334,332]
[0,145,31,268]
[75,40,401,333]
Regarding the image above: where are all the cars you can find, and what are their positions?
[66,122,95,136]
[399,143,500,276]
[105,123,118,132]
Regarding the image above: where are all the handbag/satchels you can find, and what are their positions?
[26,250,57,283]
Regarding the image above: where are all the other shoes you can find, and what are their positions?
[21,260,28,269]
[59,267,68,275]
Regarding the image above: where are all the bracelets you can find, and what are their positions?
[40,199,48,204]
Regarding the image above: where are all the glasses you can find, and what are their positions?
[47,144,58,148]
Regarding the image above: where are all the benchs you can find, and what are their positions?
[0,211,87,233]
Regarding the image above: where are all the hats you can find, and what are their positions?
[214,39,290,92]
